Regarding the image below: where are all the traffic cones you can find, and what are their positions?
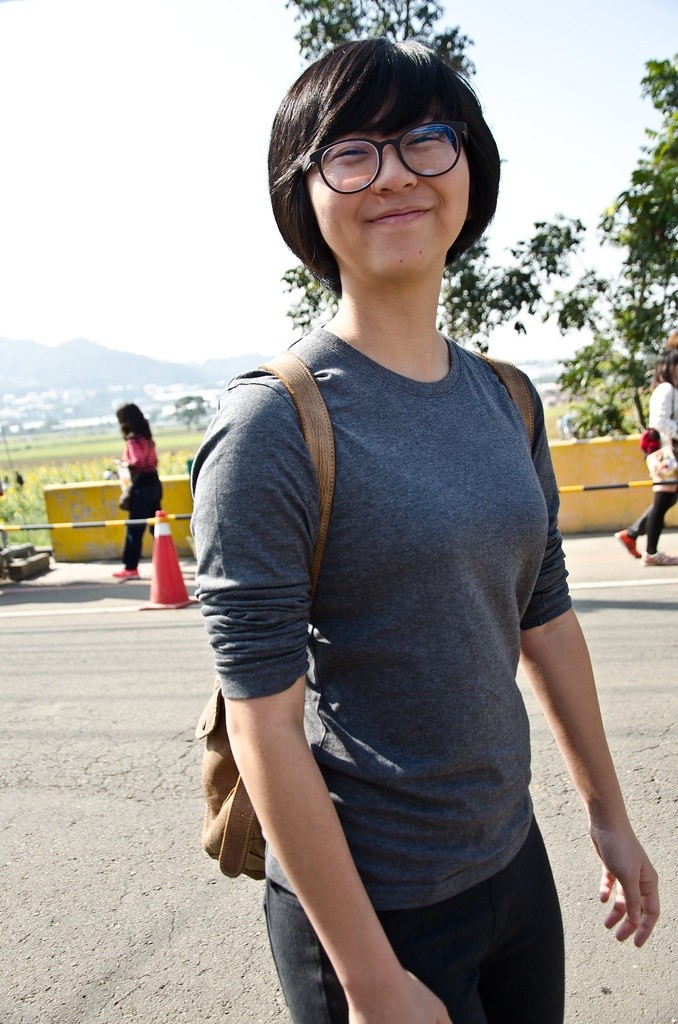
[138,510,200,611]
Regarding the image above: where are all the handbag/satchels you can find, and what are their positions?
[640,429,661,453]
[645,444,678,492]
[118,474,153,513]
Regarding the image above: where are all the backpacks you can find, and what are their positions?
[197,348,536,880]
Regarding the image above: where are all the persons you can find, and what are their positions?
[191,40,659,1024]
[112,404,163,580]
[615,332,678,567]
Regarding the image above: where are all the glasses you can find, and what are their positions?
[301,122,466,195]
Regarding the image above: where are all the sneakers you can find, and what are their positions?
[644,551,678,565]
[614,529,641,558]
[113,567,139,578]
